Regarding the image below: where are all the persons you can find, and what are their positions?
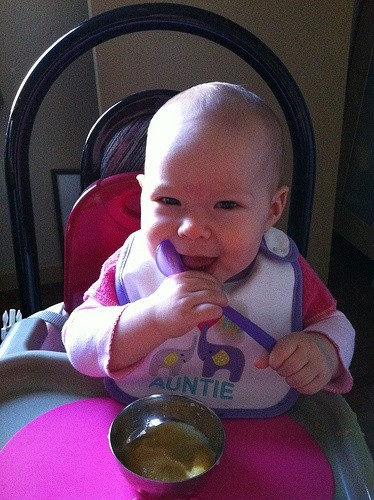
[58,81,357,422]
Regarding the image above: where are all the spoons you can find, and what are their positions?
[155,240,277,352]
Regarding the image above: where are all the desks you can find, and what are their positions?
[0,317,374,500]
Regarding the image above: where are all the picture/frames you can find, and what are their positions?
[51,167,81,270]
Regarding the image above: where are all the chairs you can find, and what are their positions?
[3,3,317,317]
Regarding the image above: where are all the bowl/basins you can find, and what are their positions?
[108,392,226,496]
